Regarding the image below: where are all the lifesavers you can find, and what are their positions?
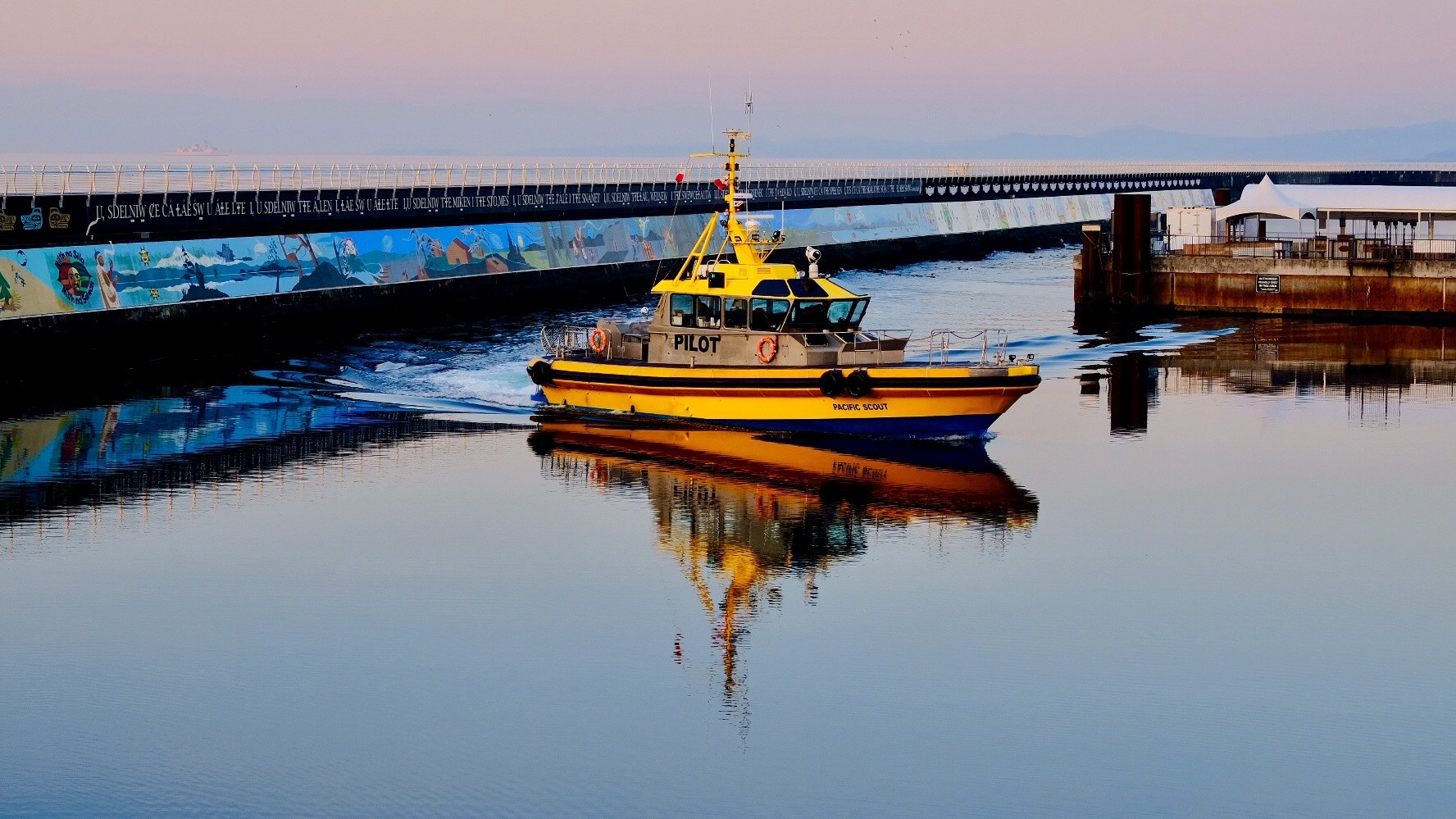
[591,464,608,484]
[756,336,776,364]
[589,327,607,353]
[531,360,553,385]
[756,492,778,521]
[819,372,843,397]
[846,372,871,398]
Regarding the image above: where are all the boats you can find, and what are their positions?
[1065,176,1456,318]
[516,90,1043,444]
